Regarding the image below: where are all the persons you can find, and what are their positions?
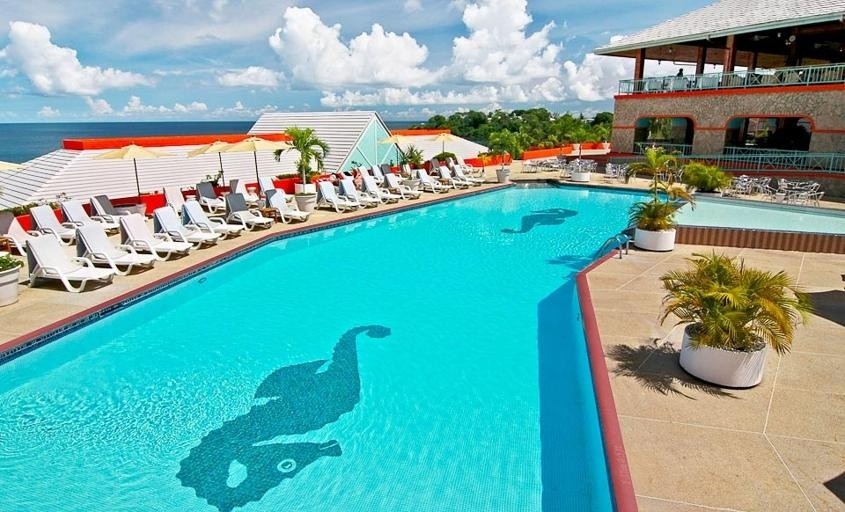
[677,68,683,77]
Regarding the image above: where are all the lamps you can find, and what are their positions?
[658,59,661,64]
[713,64,716,69]
[668,45,674,53]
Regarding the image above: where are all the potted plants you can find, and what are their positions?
[273,125,330,214]
[656,247,818,390]
[682,159,733,197]
[624,141,698,251]
[0,252,24,307]
[403,145,424,178]
[487,128,525,183]
[562,122,607,182]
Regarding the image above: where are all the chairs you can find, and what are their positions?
[518,156,685,179]
[727,171,826,209]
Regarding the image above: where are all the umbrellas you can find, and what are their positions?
[185,139,233,188]
[378,133,415,166]
[92,143,177,195]
[222,135,297,192]
[423,132,458,155]
